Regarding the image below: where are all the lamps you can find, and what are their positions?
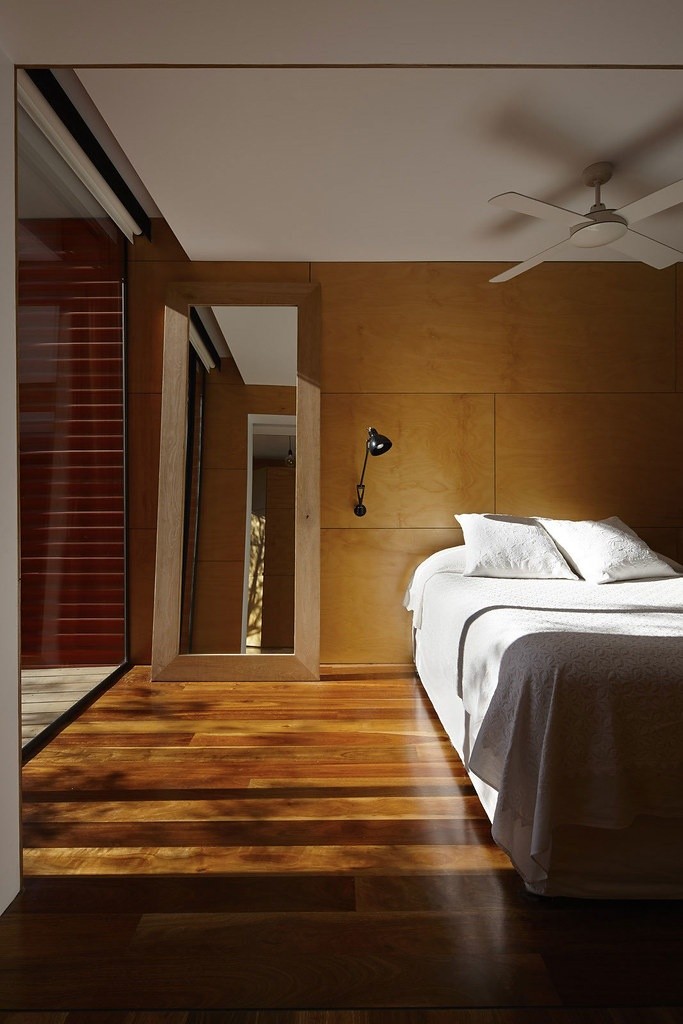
[285,436,296,467]
[353,427,393,518]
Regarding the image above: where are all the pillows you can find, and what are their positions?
[455,512,579,581]
[534,515,683,586]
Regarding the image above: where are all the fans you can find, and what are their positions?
[488,164,683,284]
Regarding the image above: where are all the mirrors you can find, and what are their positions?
[148,281,323,684]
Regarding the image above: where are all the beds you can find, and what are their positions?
[401,545,683,902]
